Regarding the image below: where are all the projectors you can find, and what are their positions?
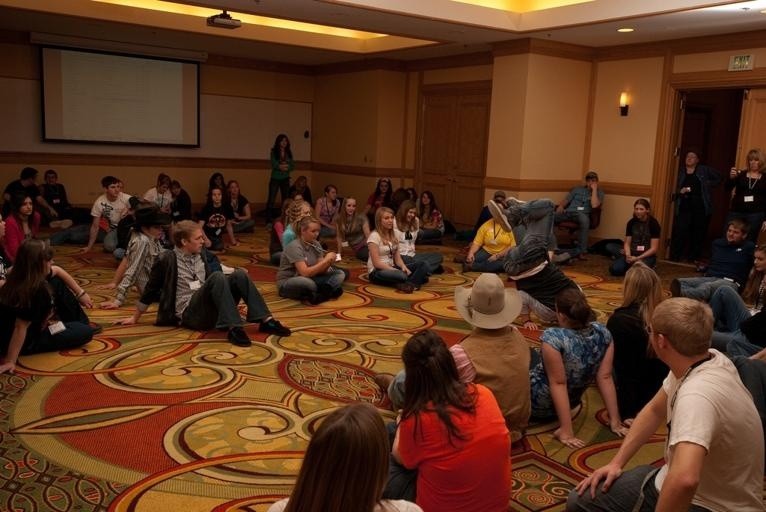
[207,16,242,30]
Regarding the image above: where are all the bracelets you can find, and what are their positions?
[76,290,86,300]
[523,320,532,323]
[496,252,501,259]
[421,224,424,230]
[232,243,237,246]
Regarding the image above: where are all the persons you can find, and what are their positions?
[416,191,445,245]
[293,191,303,204]
[113,196,170,259]
[332,193,371,262]
[392,200,443,277]
[269,199,294,266]
[108,219,291,347]
[606,262,672,427]
[565,297,765,512]
[226,180,256,233]
[0,214,13,288]
[529,288,630,450]
[207,173,226,202]
[98,207,249,310]
[78,176,133,254]
[276,216,346,306]
[725,339,765,418]
[360,177,395,230]
[467,191,506,247]
[375,273,532,444]
[166,180,192,221]
[315,184,340,237]
[487,198,597,332]
[382,327,513,512]
[663,149,724,262]
[671,218,755,304]
[725,149,766,243]
[709,284,766,350]
[281,201,311,252]
[406,186,418,202]
[2,193,41,264]
[36,169,91,229]
[198,187,240,251]
[0,167,58,220]
[268,403,424,512]
[0,239,93,376]
[69,180,124,243]
[288,176,312,206]
[464,200,516,273]
[743,244,766,316]
[144,173,173,220]
[389,187,410,215]
[553,171,604,260]
[608,199,661,277]
[366,207,431,294]
[265,134,294,228]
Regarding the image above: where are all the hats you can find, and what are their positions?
[454,272,523,330]
[134,207,171,225]
[495,191,506,199]
[586,172,598,179]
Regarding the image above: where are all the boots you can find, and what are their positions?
[311,283,334,303]
[330,286,344,299]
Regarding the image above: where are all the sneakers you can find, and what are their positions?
[577,254,587,260]
[228,326,251,347]
[87,321,101,333]
[505,197,528,208]
[488,200,518,232]
[259,321,291,336]
[396,282,414,293]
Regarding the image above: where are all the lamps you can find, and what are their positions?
[618,92,628,116]
